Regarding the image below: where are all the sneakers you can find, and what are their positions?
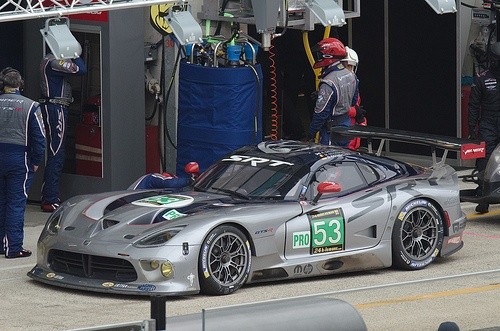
[8,249,32,258]
[474,202,490,214]
[1,247,7,255]
[40,201,63,212]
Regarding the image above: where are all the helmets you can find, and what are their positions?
[340,45,360,67]
[310,37,346,69]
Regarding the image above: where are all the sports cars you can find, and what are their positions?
[27,127,486,297]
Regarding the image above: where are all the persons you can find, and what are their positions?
[306,38,368,151]
[467,42,500,214]
[0,66,46,259]
[40,28,86,212]
[123,171,189,190]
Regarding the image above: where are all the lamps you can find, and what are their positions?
[304,0,347,28]
[424,0,457,14]
[40,13,83,61]
[162,0,204,45]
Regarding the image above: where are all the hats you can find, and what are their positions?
[490,42,500,61]
